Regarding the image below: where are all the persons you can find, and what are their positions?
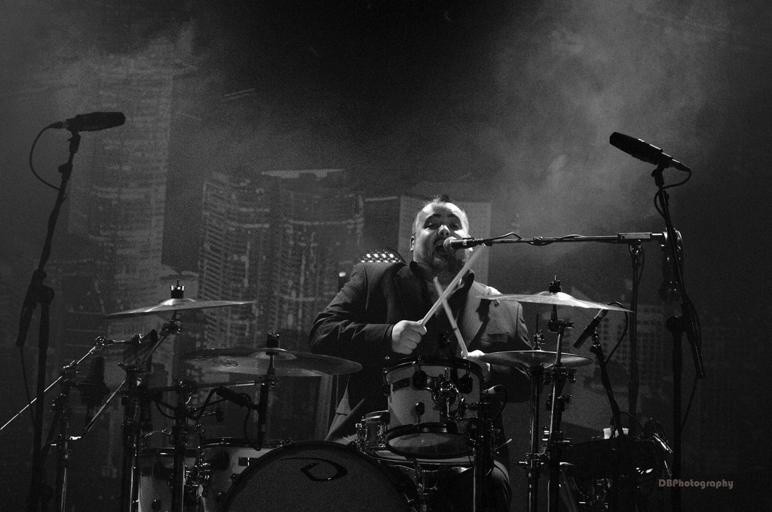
[308,193,533,512]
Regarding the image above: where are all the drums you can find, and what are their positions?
[360,357,494,466]
[128,441,420,512]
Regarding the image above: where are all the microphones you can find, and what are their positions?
[64,111,126,132]
[443,236,492,253]
[215,386,259,413]
[574,308,608,349]
[610,131,690,173]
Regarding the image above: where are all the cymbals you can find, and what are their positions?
[476,292,633,313]
[179,346,362,379]
[107,296,262,315]
[483,348,595,369]
